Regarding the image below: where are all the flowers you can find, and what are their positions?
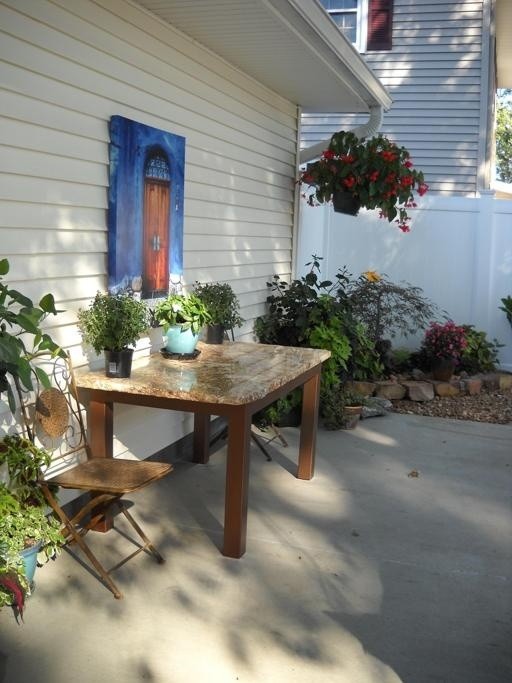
[295,130,428,233]
[423,319,469,366]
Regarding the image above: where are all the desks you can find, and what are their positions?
[71,337,333,559]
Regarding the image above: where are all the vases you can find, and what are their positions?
[333,188,362,215]
[434,366,453,382]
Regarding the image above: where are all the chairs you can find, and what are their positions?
[11,350,175,599]
[209,326,288,461]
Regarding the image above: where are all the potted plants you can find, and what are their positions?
[192,281,246,345]
[0,481,66,624]
[252,320,370,431]
[77,290,151,379]
[148,294,214,360]
